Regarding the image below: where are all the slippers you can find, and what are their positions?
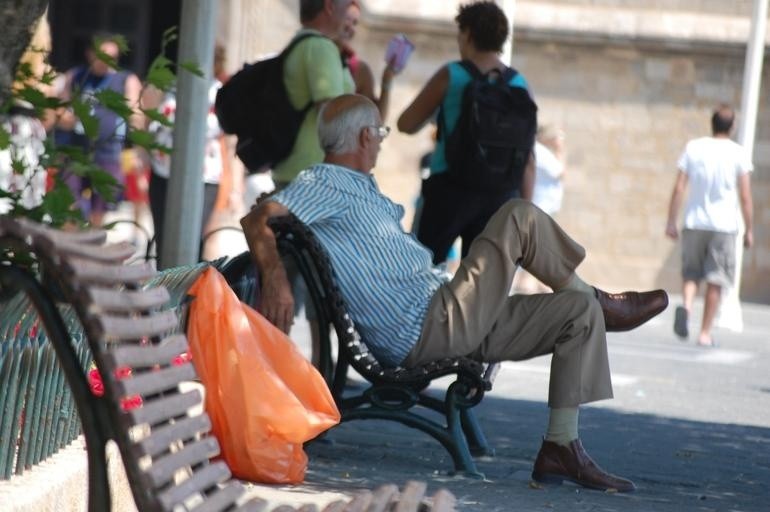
[699,339,719,348]
[674,306,688,338]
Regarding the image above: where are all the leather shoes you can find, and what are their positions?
[595,288,668,333]
[531,434,637,492]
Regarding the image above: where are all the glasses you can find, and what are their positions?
[368,126,390,137]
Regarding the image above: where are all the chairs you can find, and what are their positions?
[249,192,502,481]
[1,216,458,511]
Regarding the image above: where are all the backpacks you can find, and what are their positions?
[212,32,347,173]
[435,58,538,195]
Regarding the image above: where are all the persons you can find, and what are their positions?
[270,0,403,191]
[53,32,145,232]
[239,92,669,494]
[145,37,232,261]
[665,101,755,351]
[517,113,565,295]
[410,129,457,273]
[397,1,538,275]
[334,1,401,123]
